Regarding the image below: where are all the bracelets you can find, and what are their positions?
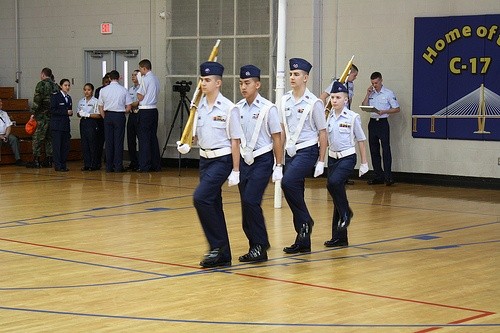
[276,164,283,166]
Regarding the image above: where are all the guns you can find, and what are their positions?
[179,37,221,151]
[324,54,356,115]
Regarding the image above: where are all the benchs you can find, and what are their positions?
[0,87,82,164]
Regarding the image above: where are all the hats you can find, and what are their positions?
[240,65,261,79]
[289,57,312,73]
[200,62,224,76]
[330,81,348,93]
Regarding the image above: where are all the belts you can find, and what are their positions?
[370,118,387,122]
[328,146,356,160]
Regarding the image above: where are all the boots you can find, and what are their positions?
[42,156,53,168]
[25,156,41,169]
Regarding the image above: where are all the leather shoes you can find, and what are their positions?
[283,244,312,254]
[199,247,232,267]
[300,218,314,238]
[323,237,349,248]
[238,244,268,262]
[336,211,354,232]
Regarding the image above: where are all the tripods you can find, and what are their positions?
[160,94,191,176]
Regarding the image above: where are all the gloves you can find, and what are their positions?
[227,169,241,187]
[176,140,192,155]
[313,160,325,178]
[271,166,284,183]
[359,162,370,178]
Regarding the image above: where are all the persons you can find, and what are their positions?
[95,77,111,168]
[362,72,400,186]
[50,79,73,171]
[324,81,370,247]
[77,83,100,171]
[176,61,242,267]
[134,59,161,171]
[320,64,358,109]
[237,64,283,262]
[126,70,139,172]
[278,58,327,252]
[25,67,60,169]
[99,70,133,172]
[0,99,26,166]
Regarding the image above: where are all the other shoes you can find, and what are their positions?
[384,178,395,187]
[369,170,383,179]
